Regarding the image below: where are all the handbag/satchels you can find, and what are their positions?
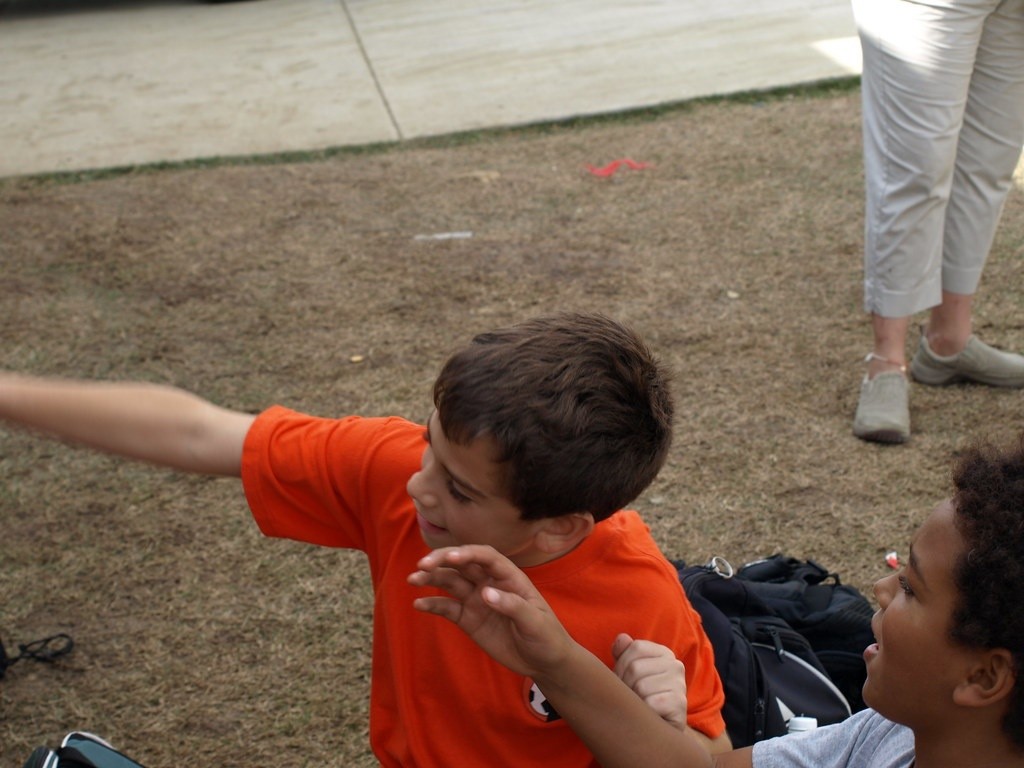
[672,544,879,745]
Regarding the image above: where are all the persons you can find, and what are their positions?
[1,314,733,768]
[409,438,1024,768]
[851,0,1024,443]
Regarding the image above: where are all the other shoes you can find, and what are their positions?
[912,334,1024,388]
[853,370,911,444]
